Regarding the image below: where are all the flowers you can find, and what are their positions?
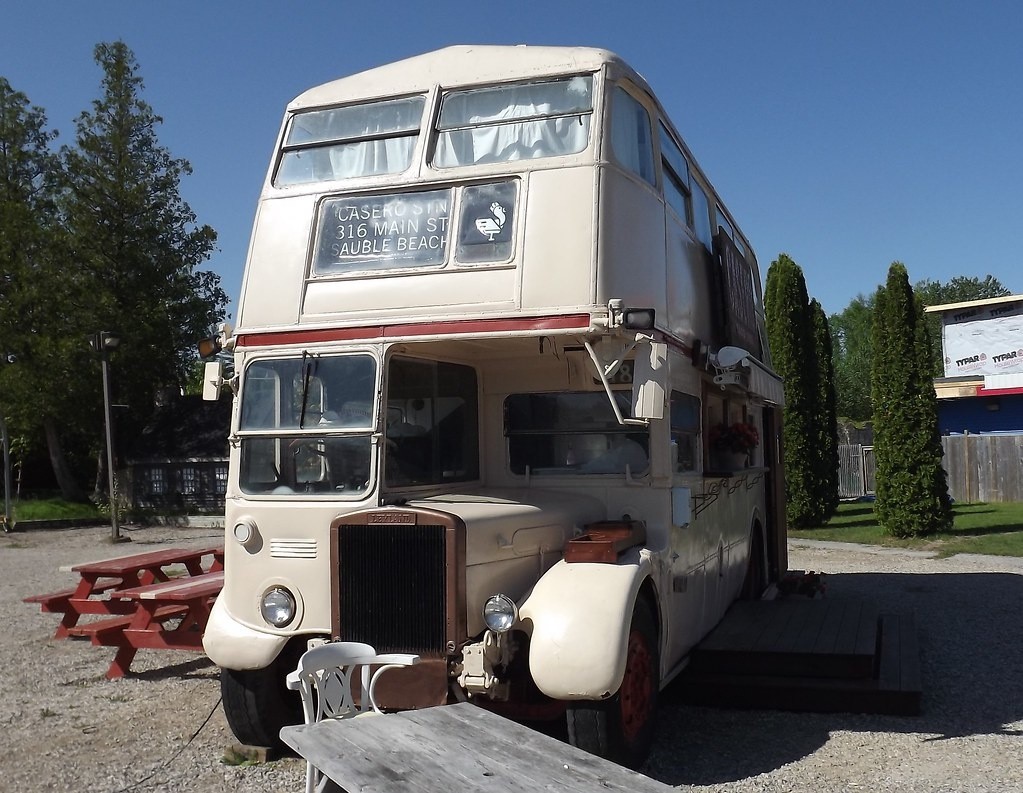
[709,421,759,455]
[791,571,828,598]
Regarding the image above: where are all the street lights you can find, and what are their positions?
[87,330,122,539]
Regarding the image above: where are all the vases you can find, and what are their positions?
[715,448,747,468]
[789,593,815,600]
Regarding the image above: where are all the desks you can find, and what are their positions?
[107,571,225,679]
[279,701,685,793]
[54,548,219,679]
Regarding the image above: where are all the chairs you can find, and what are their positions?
[286,641,421,793]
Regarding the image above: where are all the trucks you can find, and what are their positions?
[201,42,790,746]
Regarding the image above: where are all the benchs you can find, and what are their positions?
[66,596,217,637]
[20,578,123,606]
